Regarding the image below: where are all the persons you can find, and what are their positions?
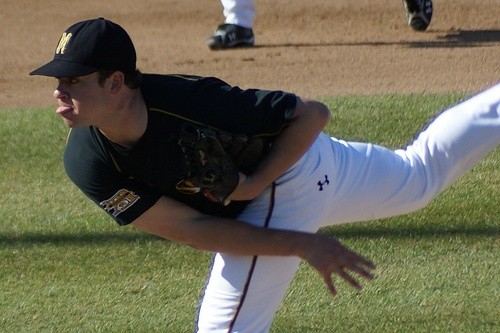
[26,16,500,333]
[206,0,434,49]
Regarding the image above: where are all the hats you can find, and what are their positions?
[28,17,136,78]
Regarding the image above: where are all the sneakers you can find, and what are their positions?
[402,0,433,33]
[207,24,255,50]
